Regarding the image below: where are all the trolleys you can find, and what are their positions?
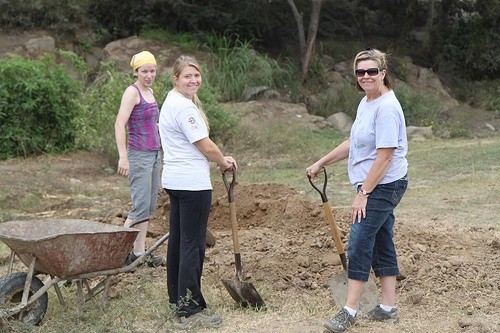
[0,216,170,326]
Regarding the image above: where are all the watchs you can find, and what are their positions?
[361,186,371,196]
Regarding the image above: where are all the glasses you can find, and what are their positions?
[355,68,384,77]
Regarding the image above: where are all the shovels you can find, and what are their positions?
[221,165,267,311]
[306,166,380,315]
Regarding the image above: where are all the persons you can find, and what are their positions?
[158,56,239,327]
[115,51,167,267]
[305,50,411,333]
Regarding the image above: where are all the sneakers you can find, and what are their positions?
[173,312,222,330]
[125,251,163,267]
[204,300,211,309]
[362,303,400,324]
[324,307,357,333]
